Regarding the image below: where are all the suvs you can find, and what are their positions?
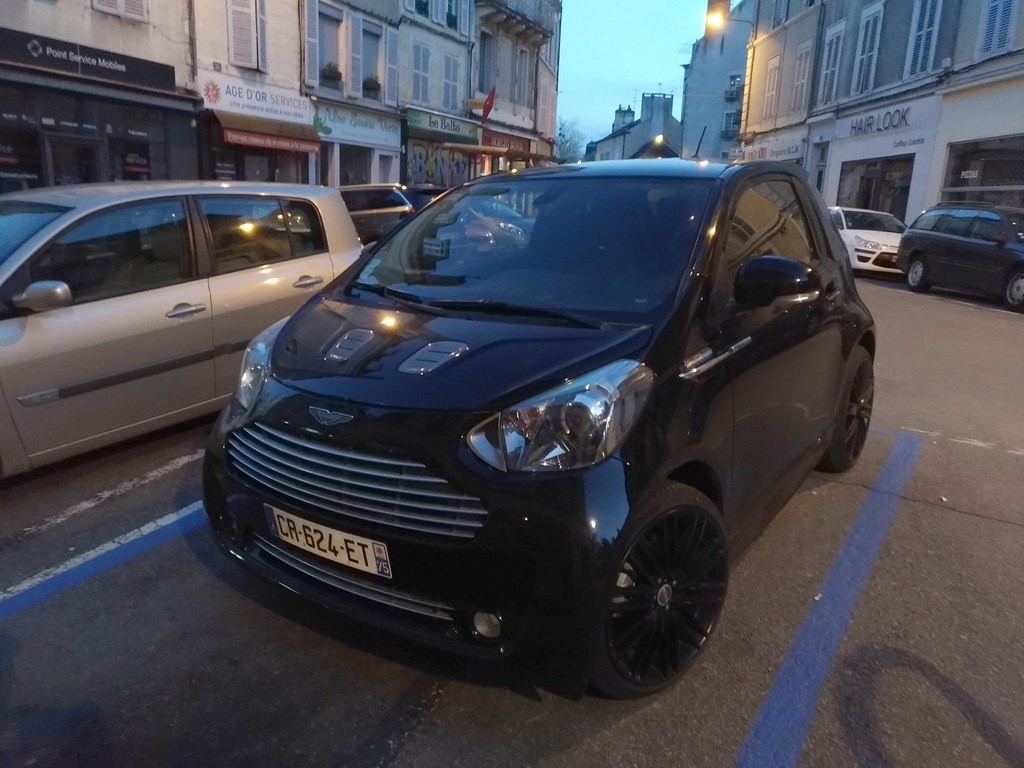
[336,184,415,245]
[896,199,1024,313]
[401,183,467,247]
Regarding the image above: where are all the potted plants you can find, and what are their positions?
[363,77,382,100]
[321,64,342,89]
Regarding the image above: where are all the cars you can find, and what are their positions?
[471,195,536,233]
[466,207,526,250]
[209,159,879,703]
[463,222,497,259]
[828,206,914,278]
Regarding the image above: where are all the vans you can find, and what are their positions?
[1,179,375,481]
